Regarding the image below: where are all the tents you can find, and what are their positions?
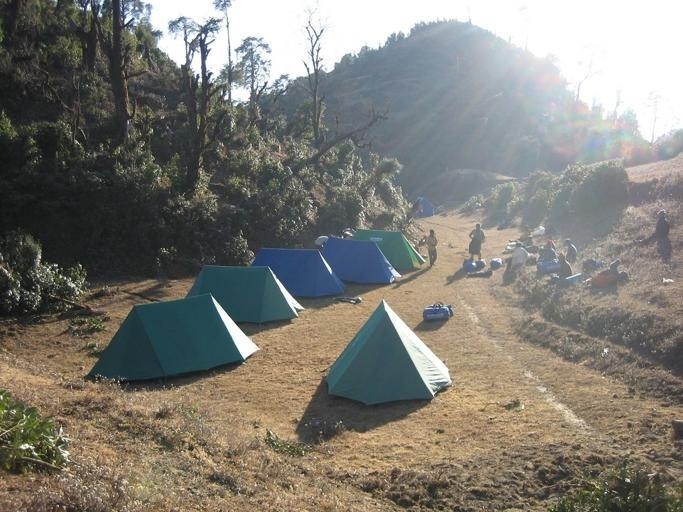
[319,237,401,283]
[350,228,425,271]
[183,264,304,324]
[82,292,258,384]
[250,247,346,298]
[410,197,437,218]
[325,301,451,406]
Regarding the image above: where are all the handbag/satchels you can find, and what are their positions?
[423,304,450,322]
[425,304,454,316]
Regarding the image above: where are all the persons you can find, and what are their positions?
[550,252,572,284]
[537,243,556,273]
[632,210,670,246]
[468,225,485,260]
[502,243,529,271]
[562,239,577,261]
[426,230,437,265]
